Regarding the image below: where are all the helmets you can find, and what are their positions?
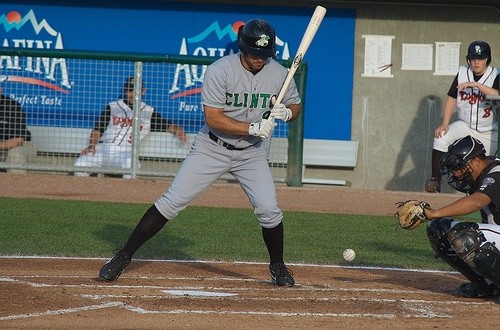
[444,135,485,171]
[467,40,491,63]
[239,19,276,57]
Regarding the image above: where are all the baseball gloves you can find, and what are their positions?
[397,200,430,230]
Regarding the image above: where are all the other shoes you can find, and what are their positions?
[460,282,489,297]
[426,181,440,193]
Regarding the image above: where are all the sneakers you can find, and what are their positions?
[268,263,295,287]
[100,248,130,281]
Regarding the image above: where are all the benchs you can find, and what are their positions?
[25,126,359,186]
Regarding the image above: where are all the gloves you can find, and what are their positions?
[269,96,293,123]
[248,118,278,139]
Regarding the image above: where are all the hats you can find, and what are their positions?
[123,77,143,91]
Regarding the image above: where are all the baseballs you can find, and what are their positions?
[342,248,356,261]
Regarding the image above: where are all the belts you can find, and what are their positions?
[208,131,242,151]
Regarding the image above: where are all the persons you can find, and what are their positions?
[73,77,187,179]
[99,19,301,286]
[0,88,31,173]
[426,41,500,194]
[396,135,500,302]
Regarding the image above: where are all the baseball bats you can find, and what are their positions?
[260,5,326,141]
